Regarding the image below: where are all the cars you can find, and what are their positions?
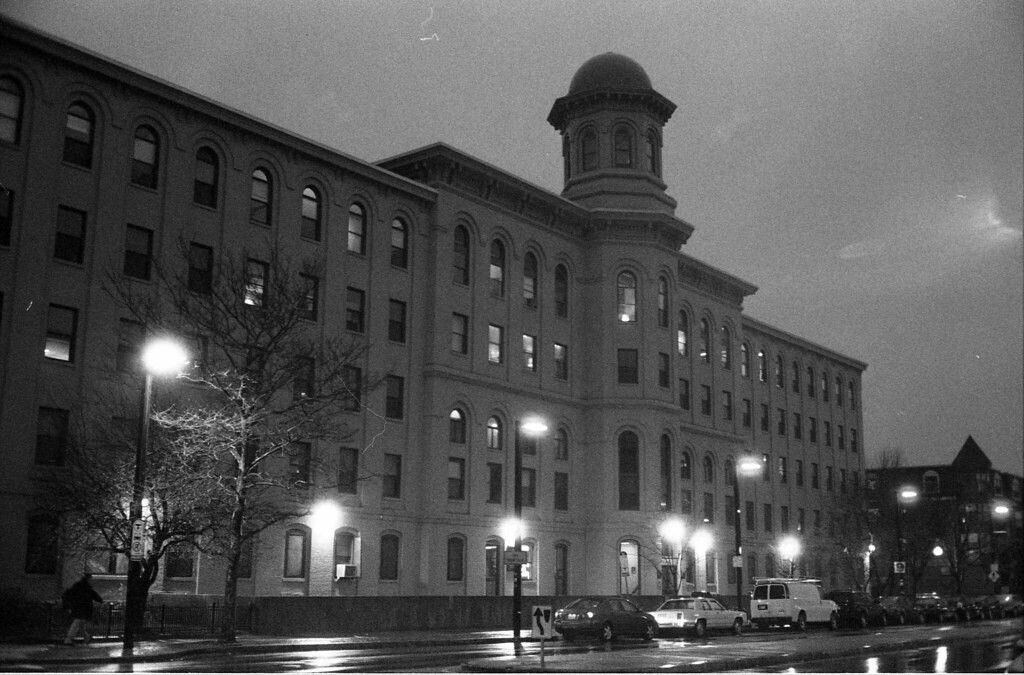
[553,596,659,643]
[646,596,750,638]
[882,594,1024,626]
[826,590,889,629]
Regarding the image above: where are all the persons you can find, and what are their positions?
[62,574,104,646]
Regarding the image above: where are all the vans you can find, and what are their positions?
[750,580,842,633]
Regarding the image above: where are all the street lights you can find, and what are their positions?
[513,416,549,642]
[734,457,762,608]
[894,484,921,593]
[125,334,190,651]
